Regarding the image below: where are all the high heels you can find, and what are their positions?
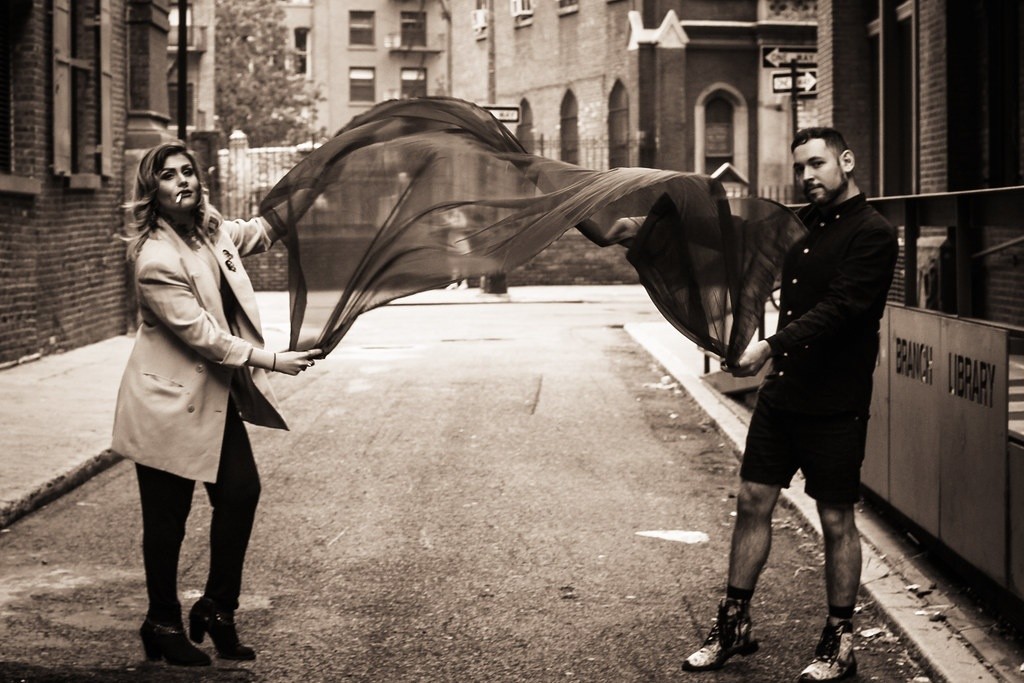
[188,597,257,661]
[139,615,211,666]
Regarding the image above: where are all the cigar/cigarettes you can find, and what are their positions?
[176,193,182,203]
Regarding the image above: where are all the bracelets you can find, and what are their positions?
[630,216,647,227]
[271,353,276,372]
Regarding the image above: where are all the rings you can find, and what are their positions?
[308,361,312,367]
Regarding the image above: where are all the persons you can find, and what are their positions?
[110,143,346,667]
[605,126,899,683]
[441,204,470,289]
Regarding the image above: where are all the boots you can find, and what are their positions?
[681,598,753,672]
[798,617,857,683]
